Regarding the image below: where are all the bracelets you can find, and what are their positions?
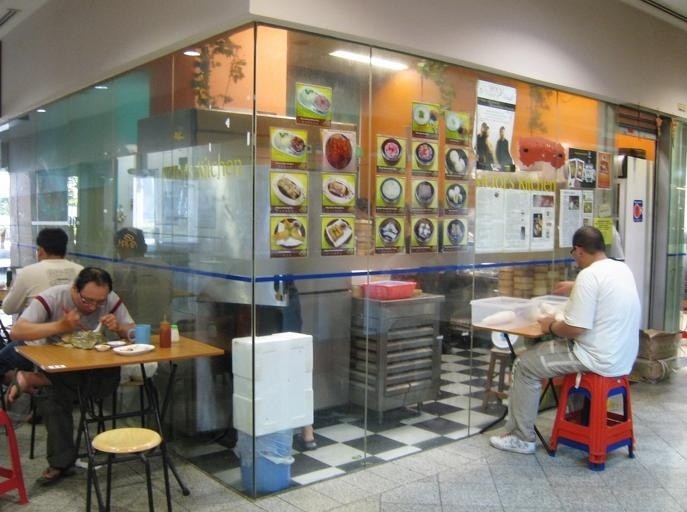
[547,319,555,334]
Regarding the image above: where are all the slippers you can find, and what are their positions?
[34,466,76,486]
[2,368,24,411]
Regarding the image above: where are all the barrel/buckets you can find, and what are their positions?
[237,429,295,495]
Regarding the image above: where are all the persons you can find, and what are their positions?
[0,226,89,386]
[475,119,494,170]
[494,125,513,171]
[488,227,640,453]
[2,265,136,485]
[101,226,176,325]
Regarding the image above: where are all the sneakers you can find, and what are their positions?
[488,432,538,455]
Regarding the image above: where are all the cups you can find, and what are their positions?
[126,322,153,345]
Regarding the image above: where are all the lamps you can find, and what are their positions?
[182,47,202,57]
[326,44,409,72]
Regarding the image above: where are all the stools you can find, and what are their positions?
[85,427,163,512]
[112,374,145,429]
[546,372,637,472]
[482,346,527,410]
[0,407,30,505]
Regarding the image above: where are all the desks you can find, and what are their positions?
[479,323,559,458]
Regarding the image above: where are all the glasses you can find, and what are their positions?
[79,292,109,309]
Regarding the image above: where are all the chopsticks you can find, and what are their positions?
[60,305,88,332]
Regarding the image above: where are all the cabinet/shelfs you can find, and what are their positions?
[349,292,446,426]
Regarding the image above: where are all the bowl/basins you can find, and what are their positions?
[68,329,104,350]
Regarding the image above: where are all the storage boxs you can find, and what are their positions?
[637,328,678,361]
[230,330,315,439]
[360,281,417,300]
[632,357,677,380]
[530,294,568,321]
[469,294,538,331]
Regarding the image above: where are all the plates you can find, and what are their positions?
[269,86,468,252]
[93,338,158,356]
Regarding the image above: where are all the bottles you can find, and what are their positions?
[160,320,180,349]
